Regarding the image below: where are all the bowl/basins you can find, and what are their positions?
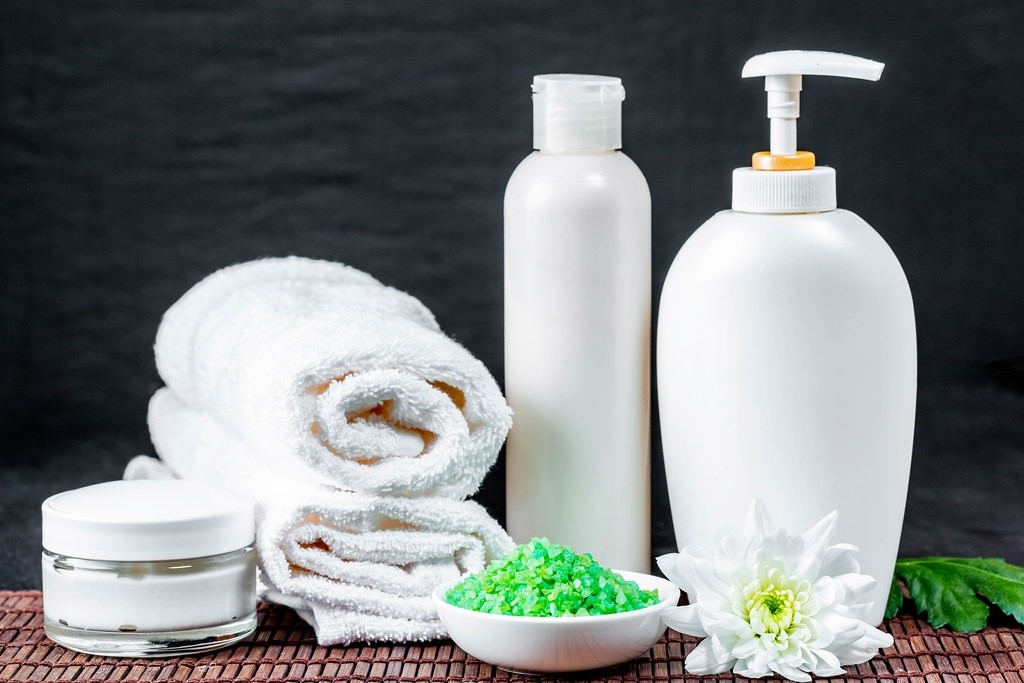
[429,568,680,675]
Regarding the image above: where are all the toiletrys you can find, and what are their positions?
[35,478,261,662]
[499,69,656,582]
[657,48,921,655]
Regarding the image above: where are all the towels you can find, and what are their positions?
[153,252,517,507]
[140,386,525,654]
